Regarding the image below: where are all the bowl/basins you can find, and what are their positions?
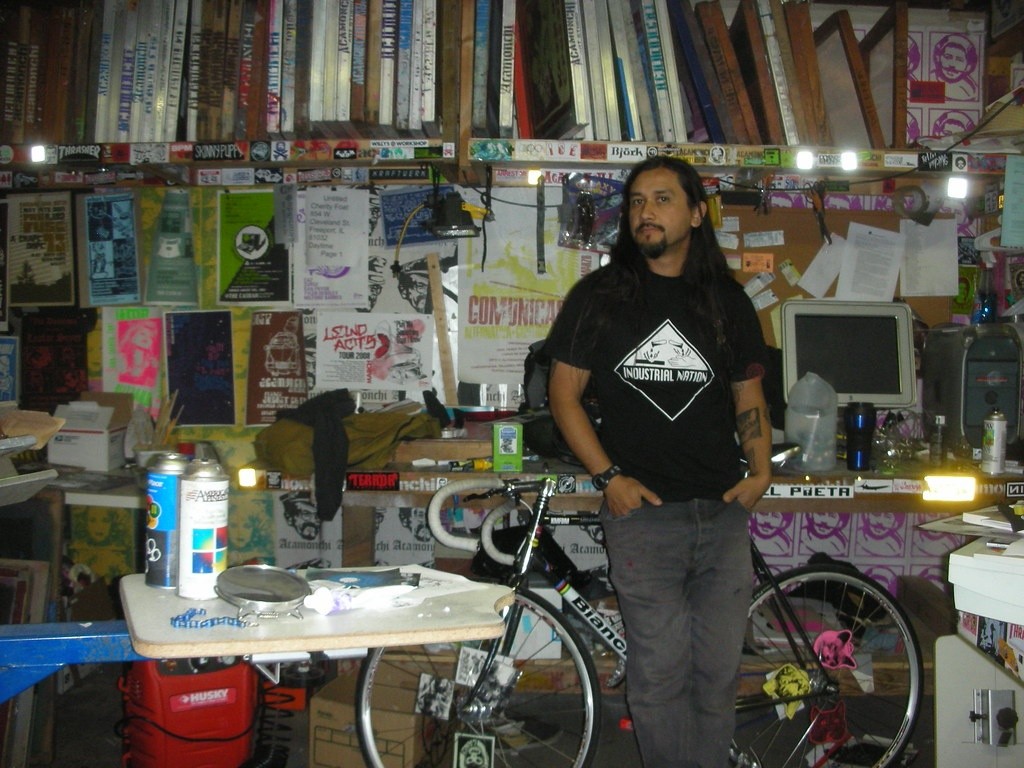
[132,447,189,491]
[788,371,838,418]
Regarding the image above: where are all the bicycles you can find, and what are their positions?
[353,470,924,768]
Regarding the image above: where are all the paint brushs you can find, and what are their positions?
[127,389,185,445]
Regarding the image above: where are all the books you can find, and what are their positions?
[962,504,1024,534]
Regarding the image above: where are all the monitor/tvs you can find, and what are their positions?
[780,300,917,409]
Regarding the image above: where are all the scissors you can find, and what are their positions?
[802,180,832,245]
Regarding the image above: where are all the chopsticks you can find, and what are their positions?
[140,388,185,450]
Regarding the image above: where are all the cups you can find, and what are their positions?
[784,409,838,472]
[844,401,877,471]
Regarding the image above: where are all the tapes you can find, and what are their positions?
[895,185,926,218]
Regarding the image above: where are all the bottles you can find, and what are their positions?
[929,415,949,467]
[303,585,425,615]
[144,452,191,590]
[981,406,1009,477]
[177,456,231,601]
[970,265,998,325]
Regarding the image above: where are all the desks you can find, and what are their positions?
[121,566,516,658]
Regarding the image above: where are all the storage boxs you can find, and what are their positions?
[310,651,461,768]
[48,392,134,473]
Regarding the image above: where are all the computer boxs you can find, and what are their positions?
[922,322,1022,458]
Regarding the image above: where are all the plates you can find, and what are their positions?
[216,564,313,613]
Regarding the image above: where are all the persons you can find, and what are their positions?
[539,157,772,768]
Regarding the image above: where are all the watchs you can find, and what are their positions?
[591,464,623,491]
